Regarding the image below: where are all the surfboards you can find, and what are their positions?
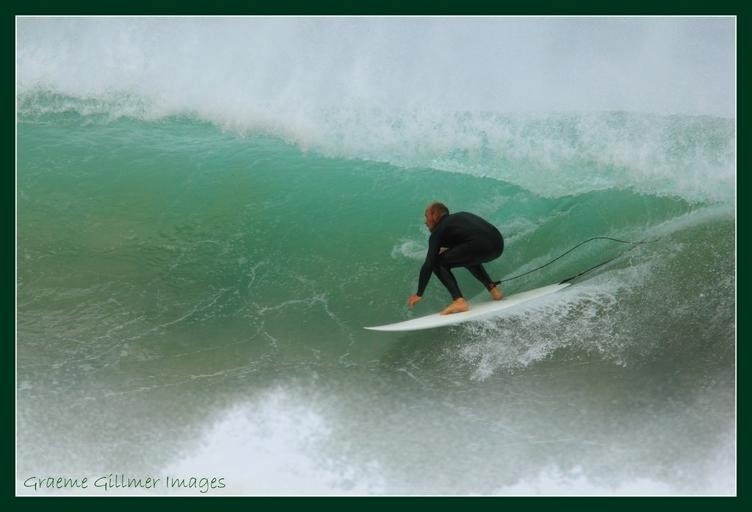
[362,282,572,331]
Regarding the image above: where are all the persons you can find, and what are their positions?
[407,202,504,316]
